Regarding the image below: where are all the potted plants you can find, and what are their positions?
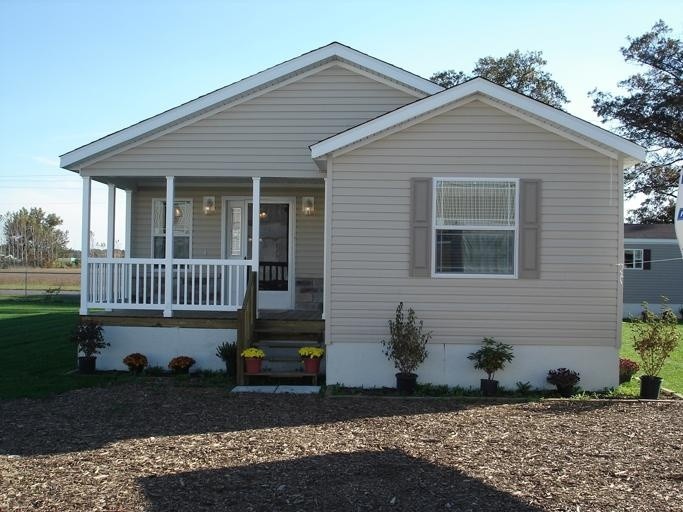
[168,357,195,374]
[70,320,107,374]
[378,302,434,396]
[467,336,514,396]
[547,367,580,398]
[216,341,237,385]
[124,353,148,373]
[620,357,639,386]
[628,295,681,399]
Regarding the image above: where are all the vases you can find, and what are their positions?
[245,358,261,374]
[304,359,320,374]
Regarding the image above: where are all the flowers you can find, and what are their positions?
[240,348,265,358]
[299,346,326,359]
